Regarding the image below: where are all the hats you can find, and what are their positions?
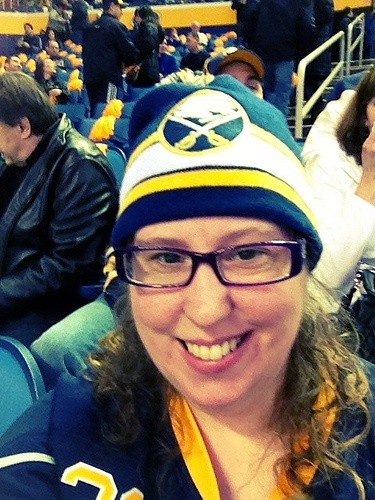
[102,0,129,10]
[111,68,323,271]
[206,45,264,80]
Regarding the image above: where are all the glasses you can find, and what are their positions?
[116,237,308,288]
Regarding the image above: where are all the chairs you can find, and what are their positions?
[0,335,46,438]
[54,85,158,191]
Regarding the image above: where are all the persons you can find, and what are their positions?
[301,66,375,361]
[1,1,356,118]
[1,71,118,387]
[1,73,375,499]
